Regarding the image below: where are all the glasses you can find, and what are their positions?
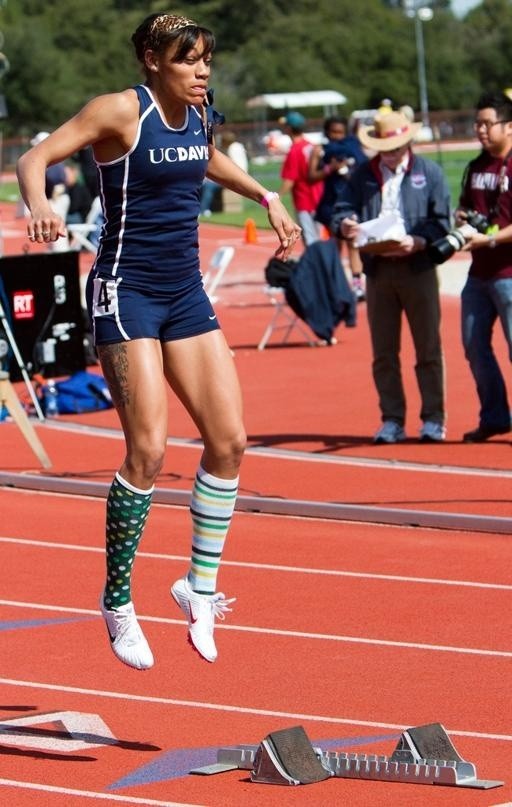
[474,120,510,129]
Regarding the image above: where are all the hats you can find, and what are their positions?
[358,111,422,151]
[279,112,305,128]
[30,132,49,146]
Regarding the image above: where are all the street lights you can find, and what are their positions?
[404,0,436,126]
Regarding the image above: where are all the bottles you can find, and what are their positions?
[44,378,60,420]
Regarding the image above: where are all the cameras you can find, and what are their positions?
[429,210,491,264]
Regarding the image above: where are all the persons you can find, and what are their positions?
[195,124,251,217]
[21,128,77,254]
[64,163,95,239]
[71,144,104,249]
[329,106,456,445]
[13,9,305,672]
[452,91,512,445]
[268,108,377,307]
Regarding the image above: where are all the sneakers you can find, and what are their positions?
[352,284,367,301]
[374,420,405,443]
[170,573,224,663]
[420,421,445,442]
[463,424,511,443]
[100,587,153,670]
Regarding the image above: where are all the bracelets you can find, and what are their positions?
[258,191,279,207]
[487,232,498,248]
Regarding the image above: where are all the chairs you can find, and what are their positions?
[257,239,357,352]
[67,195,103,257]
[200,246,235,301]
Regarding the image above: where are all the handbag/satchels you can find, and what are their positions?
[266,257,297,286]
[39,373,114,414]
[34,331,99,378]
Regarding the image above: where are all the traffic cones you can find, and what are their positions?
[244,217,258,244]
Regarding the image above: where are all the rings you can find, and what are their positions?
[35,232,43,238]
[27,234,35,238]
[42,230,51,238]
